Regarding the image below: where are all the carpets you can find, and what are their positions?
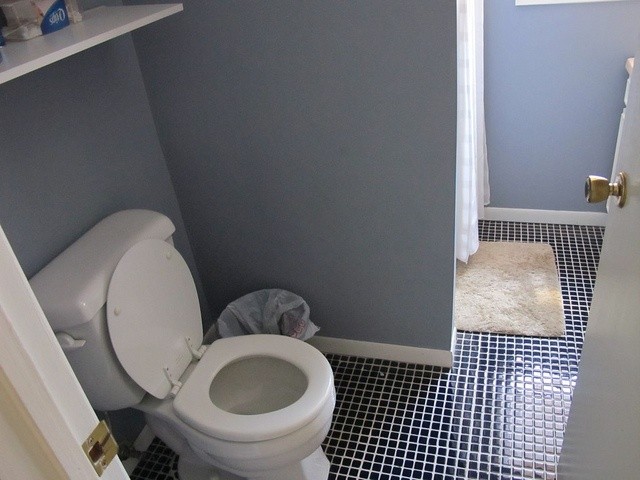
[454,240,566,338]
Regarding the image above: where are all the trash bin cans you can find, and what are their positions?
[216,288,311,339]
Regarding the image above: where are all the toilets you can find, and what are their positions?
[28,208,336,480]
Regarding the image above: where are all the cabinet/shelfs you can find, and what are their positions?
[0,2,184,85]
